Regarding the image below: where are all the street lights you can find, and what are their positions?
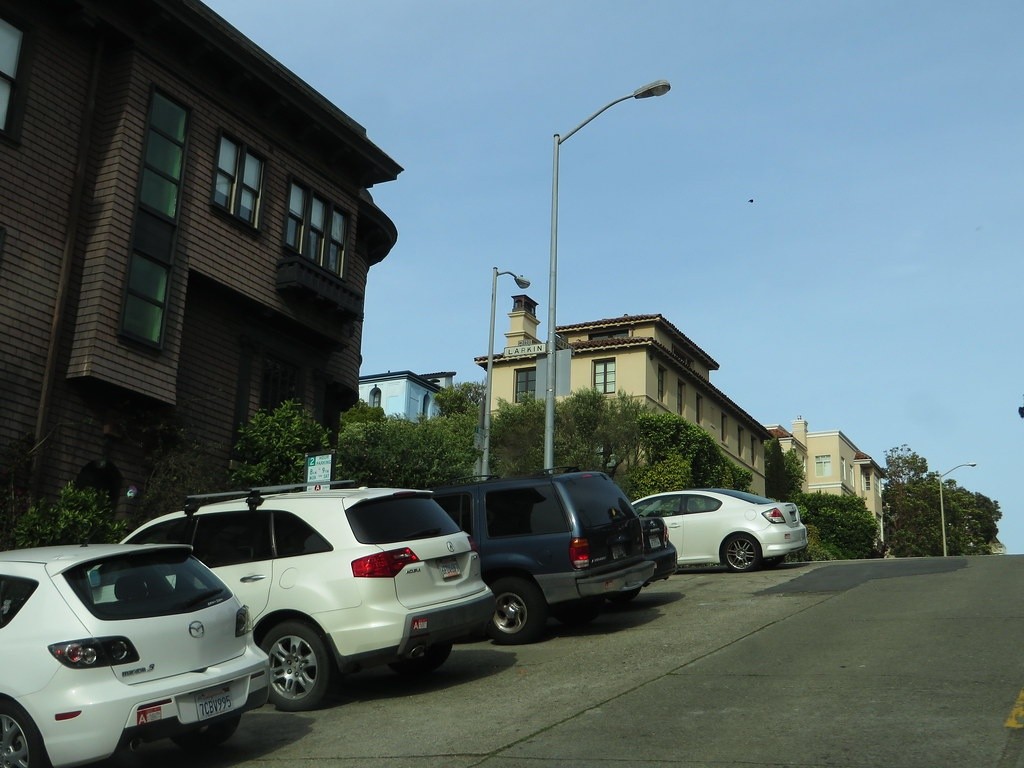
[479,266,531,479]
[940,463,977,558]
[543,79,671,474]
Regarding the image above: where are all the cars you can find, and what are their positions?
[623,486,810,584]
[0,542,272,768]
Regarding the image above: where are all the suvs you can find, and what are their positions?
[417,465,657,644]
[86,480,499,713]
